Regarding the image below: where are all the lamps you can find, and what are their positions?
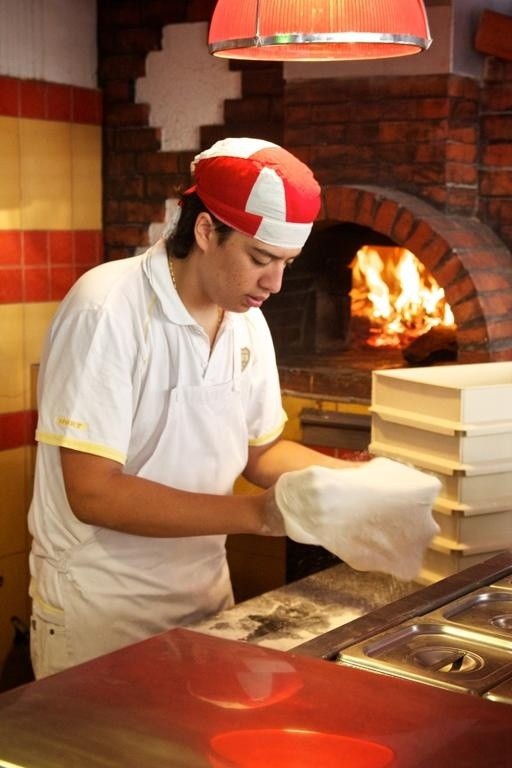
[204,2,436,64]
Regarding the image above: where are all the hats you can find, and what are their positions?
[191,138,323,250]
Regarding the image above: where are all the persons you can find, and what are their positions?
[24,136,375,688]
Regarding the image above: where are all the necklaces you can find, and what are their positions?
[168,257,223,343]
